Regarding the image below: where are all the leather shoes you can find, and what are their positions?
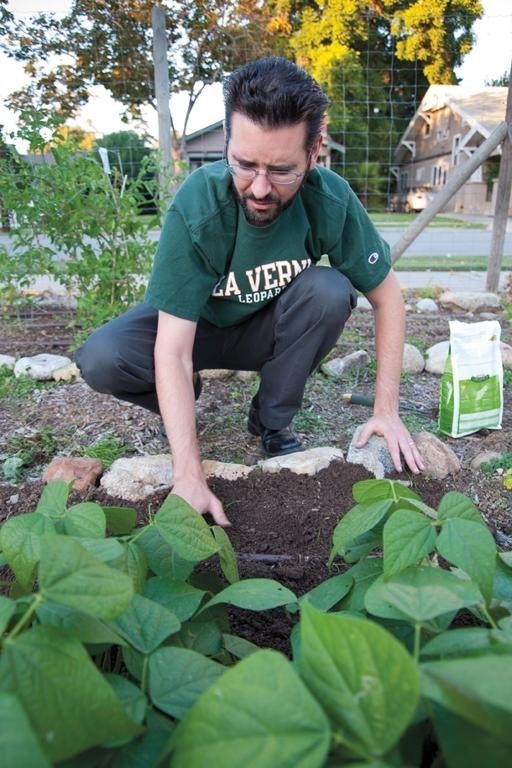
[247,399,305,456]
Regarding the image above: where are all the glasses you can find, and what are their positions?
[219,133,319,188]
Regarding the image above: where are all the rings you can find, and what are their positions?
[409,441,414,445]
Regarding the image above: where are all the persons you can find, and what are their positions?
[75,55,426,528]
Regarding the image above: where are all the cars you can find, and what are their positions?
[387,186,438,212]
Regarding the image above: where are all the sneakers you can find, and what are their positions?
[159,375,203,441]
